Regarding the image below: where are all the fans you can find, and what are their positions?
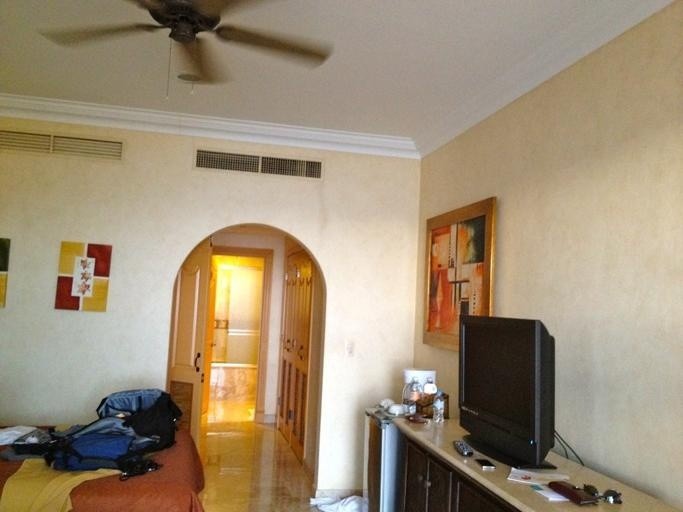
[35,0,345,87]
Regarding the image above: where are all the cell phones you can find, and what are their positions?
[475,458,497,470]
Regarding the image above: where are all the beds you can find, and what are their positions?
[0,411,204,511]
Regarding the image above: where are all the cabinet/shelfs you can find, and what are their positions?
[362,411,517,511]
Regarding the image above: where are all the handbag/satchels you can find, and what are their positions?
[96,390,183,450]
[46,432,135,471]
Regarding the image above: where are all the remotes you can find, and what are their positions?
[549,478,596,504]
[452,438,472,456]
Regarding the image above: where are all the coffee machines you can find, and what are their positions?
[401,369,436,393]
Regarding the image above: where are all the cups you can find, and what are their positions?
[379,398,404,416]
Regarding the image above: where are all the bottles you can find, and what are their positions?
[402,376,445,422]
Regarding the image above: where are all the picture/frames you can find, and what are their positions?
[419,196,500,356]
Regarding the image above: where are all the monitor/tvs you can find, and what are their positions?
[458,312,558,468]
